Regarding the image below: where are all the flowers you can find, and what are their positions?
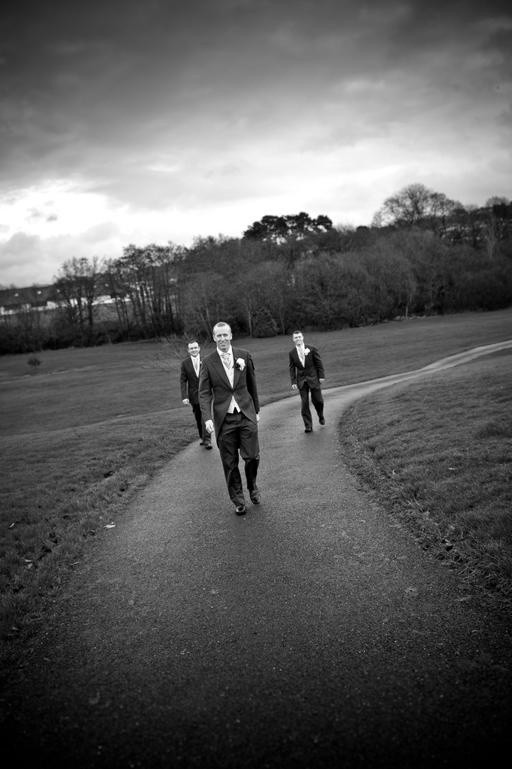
[233,356,246,372]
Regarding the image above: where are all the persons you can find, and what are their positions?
[178,340,215,449]
[198,322,261,515]
[287,330,326,433]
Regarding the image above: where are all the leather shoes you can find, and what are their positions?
[235,504,246,515]
[248,485,260,504]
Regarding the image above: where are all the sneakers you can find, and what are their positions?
[200,439,204,445]
[305,426,313,433]
[319,415,325,425]
[205,443,213,450]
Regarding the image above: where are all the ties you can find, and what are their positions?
[223,353,231,369]
[299,348,304,361]
[194,359,198,370]
